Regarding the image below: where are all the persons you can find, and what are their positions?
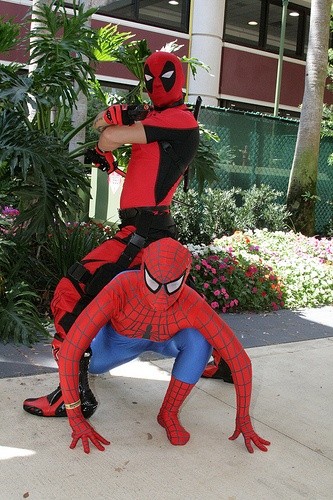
[23,47,241,421]
[58,236,272,455]
